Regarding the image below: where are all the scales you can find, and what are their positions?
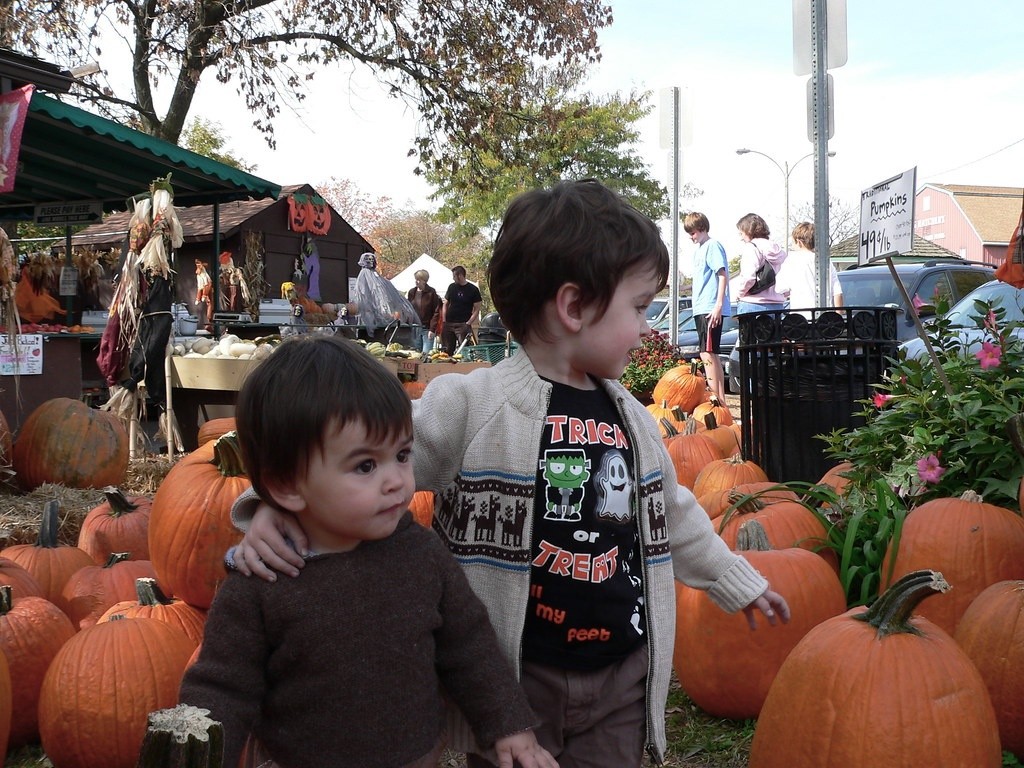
[212,311,252,323]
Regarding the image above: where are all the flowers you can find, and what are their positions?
[621,329,709,394]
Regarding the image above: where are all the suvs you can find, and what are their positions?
[729,259,997,394]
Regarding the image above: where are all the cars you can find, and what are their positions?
[897,279,1024,370]
[645,295,740,384]
[477,312,508,343]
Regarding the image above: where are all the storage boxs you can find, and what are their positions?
[168,340,522,390]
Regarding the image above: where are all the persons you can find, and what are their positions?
[409,270,442,352]
[776,222,843,319]
[442,266,482,355]
[667,213,728,402]
[178,335,561,767]
[232,182,789,767]
[729,212,789,393]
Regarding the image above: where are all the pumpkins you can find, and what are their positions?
[170,333,274,360]
[293,297,357,314]
[0,396,432,768]
[401,380,426,399]
[644,359,1024,768]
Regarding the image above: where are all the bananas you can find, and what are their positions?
[366,341,403,357]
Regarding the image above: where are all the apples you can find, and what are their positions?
[0,322,94,333]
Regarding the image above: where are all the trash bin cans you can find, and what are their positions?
[227,322,282,347]
[732,305,904,510]
[359,323,423,351]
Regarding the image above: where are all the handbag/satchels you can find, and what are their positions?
[748,242,776,294]
[994,212,1024,290]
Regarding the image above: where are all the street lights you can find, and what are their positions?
[737,149,836,257]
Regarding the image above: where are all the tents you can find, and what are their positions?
[392,253,482,323]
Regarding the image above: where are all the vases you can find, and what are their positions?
[632,388,651,400]
